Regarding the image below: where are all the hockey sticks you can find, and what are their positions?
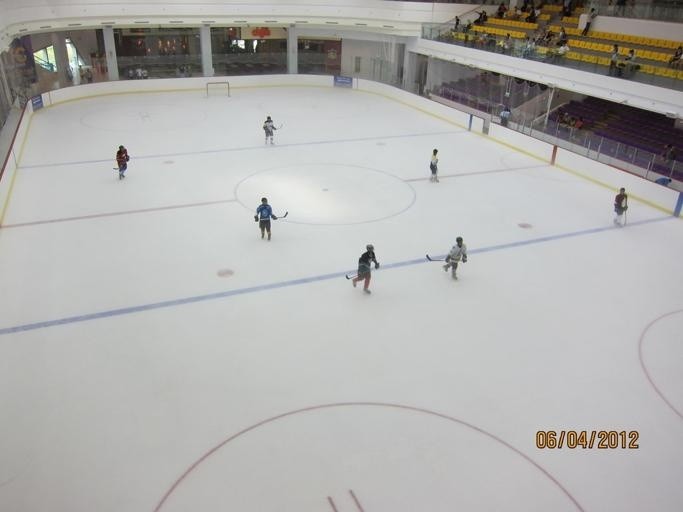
[346,267,376,279]
[258,212,288,220]
[426,254,463,262]
[622,196,627,226]
[275,124,283,130]
[113,160,127,170]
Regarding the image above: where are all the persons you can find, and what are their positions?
[444,237,467,280]
[353,245,379,294]
[660,144,677,162]
[429,149,440,183]
[563,113,584,129]
[614,188,628,226]
[86,69,93,83]
[265,116,276,144]
[257,197,277,240]
[455,0,683,79]
[500,107,511,128]
[128,67,148,80]
[116,146,127,180]
[655,177,672,186]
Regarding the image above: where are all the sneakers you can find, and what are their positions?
[363,288,371,295]
[452,273,458,280]
[614,219,621,226]
[119,174,125,181]
[265,140,275,144]
[431,177,440,183]
[261,232,271,240]
[442,264,450,272]
[353,279,356,287]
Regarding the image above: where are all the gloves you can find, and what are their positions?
[462,256,467,263]
[254,216,259,222]
[446,257,451,263]
[271,215,278,221]
[375,263,380,269]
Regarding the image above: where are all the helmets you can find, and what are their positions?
[367,245,374,250]
[456,237,463,242]
